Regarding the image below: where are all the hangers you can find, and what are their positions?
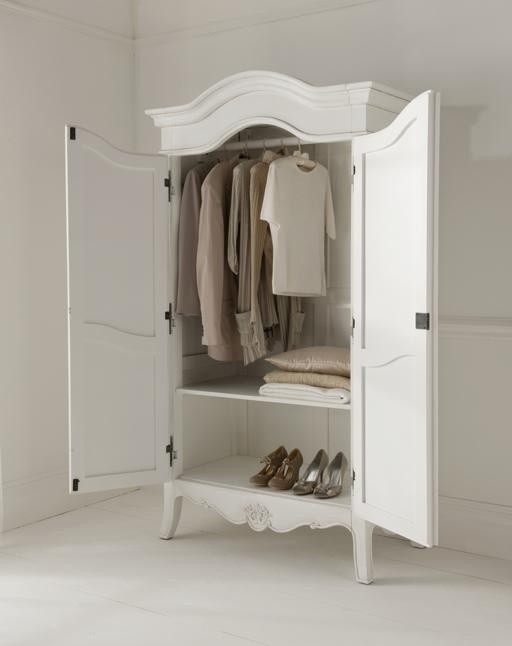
[221,135,330,169]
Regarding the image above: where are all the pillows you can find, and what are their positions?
[265,347,351,376]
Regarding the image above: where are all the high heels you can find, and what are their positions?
[313,452,347,498]
[249,446,304,490]
[293,450,329,494]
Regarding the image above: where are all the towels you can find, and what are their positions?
[257,382,349,404]
[263,370,350,389]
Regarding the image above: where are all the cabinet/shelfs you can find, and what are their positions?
[64,72,444,584]
